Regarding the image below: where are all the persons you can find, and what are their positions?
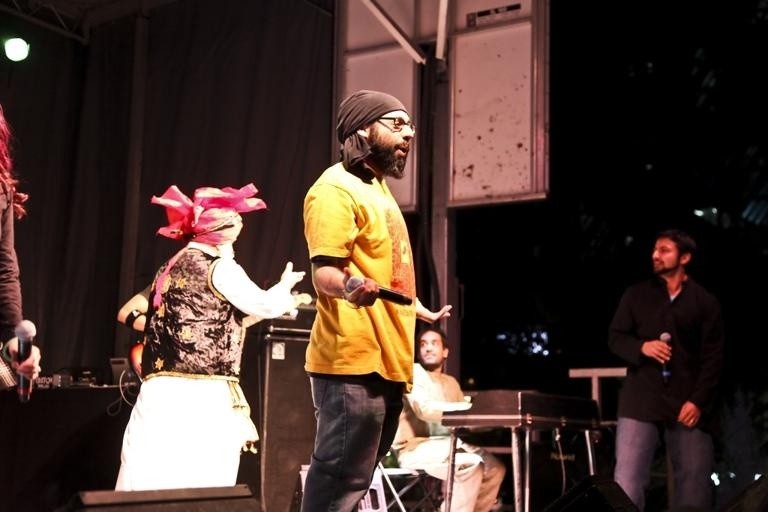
[609,224,729,512]
[300,84,456,511]
[390,326,506,512]
[111,182,307,494]
[1,108,44,389]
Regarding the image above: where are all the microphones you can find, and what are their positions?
[345,275,412,306]
[14,319,37,404]
[659,332,672,384]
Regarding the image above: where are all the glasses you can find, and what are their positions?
[380,116,416,134]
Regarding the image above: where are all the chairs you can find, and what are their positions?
[376,460,443,512]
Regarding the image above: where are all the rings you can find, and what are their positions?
[689,419,695,424]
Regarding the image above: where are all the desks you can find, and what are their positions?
[444,419,593,512]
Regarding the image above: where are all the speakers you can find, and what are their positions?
[237,333,319,512]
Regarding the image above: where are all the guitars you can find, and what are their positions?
[128,294,313,388]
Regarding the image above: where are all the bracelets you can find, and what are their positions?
[2,335,21,363]
[340,288,364,311]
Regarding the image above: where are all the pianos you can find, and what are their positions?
[438,388,600,431]
